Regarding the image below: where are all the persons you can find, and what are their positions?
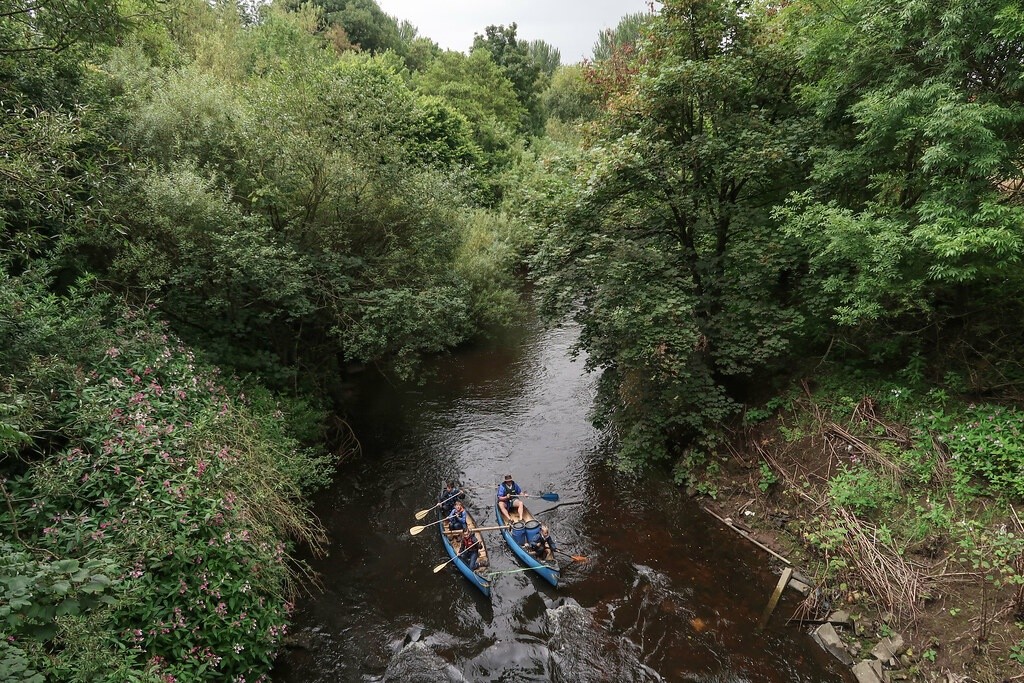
[527,524,560,564]
[496,474,529,523]
[437,481,489,569]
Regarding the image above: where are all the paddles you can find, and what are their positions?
[415,491,461,521]
[554,549,588,562]
[433,540,477,574]
[510,493,559,501]
[409,515,457,535]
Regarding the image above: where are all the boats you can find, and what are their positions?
[495,484,561,587]
[437,480,491,598]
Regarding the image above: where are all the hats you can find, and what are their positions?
[504,475,513,481]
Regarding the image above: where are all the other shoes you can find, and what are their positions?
[518,519,524,524]
[509,519,514,525]
[479,560,485,566]
[455,536,462,543]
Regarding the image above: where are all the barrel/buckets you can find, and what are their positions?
[511,521,526,545]
[525,520,541,543]
